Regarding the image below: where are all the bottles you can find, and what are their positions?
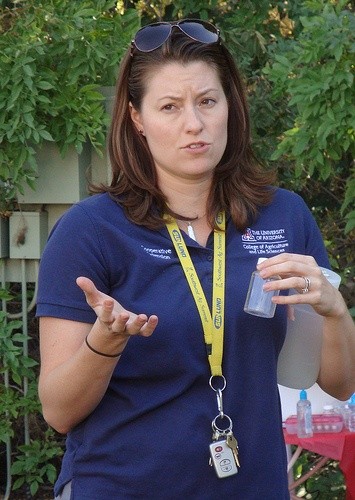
[244,257,282,318]
[297,389,313,438]
[276,266,341,391]
[323,393,355,432]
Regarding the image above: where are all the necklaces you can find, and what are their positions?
[185,213,207,241]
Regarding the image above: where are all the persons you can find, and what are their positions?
[36,20,355,499]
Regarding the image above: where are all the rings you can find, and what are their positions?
[299,275,311,294]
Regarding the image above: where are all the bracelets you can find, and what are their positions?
[85,336,123,357]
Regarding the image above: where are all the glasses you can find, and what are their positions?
[132,18,222,51]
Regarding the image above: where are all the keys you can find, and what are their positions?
[209,429,241,469]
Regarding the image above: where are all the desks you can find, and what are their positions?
[282,424,354,500]
